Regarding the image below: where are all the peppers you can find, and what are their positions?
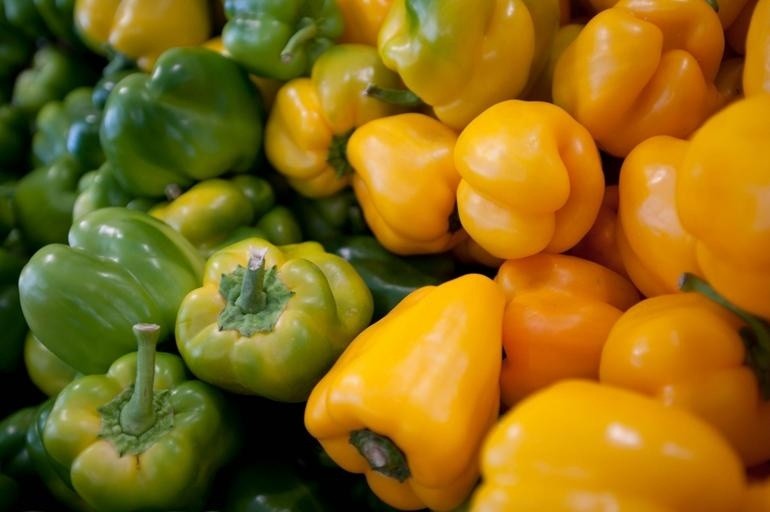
[2,0,769,511]
